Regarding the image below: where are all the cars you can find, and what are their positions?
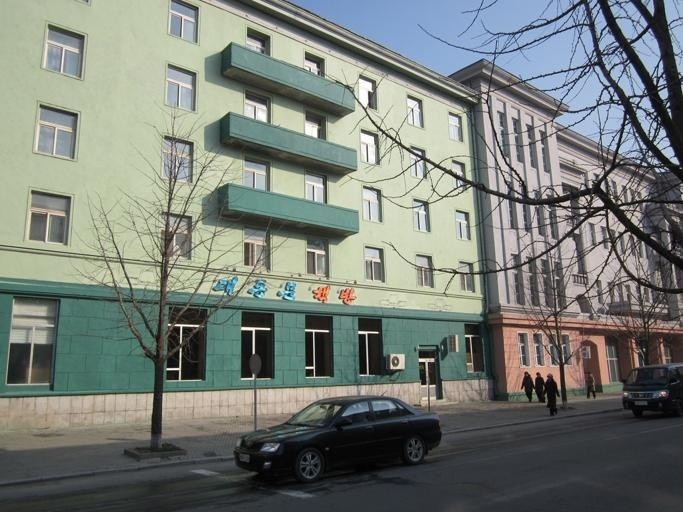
[233,395,442,483]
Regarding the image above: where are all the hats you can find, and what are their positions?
[547,374,553,378]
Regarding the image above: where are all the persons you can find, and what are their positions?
[542,373,560,416]
[585,372,596,399]
[534,373,545,403]
[658,368,671,383]
[520,372,535,403]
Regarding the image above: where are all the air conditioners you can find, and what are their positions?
[385,354,406,373]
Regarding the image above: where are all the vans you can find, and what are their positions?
[622,364,682,419]
